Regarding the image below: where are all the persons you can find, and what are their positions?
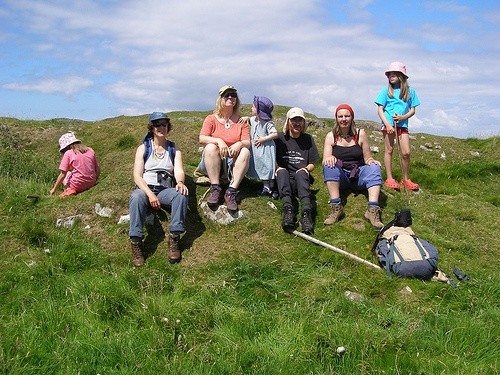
[50,133,99,197]
[129,111,188,266]
[321,103,384,227]
[198,85,250,210]
[375,61,420,190]
[237,95,278,193]
[273,107,317,233]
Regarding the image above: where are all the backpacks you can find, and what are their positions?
[376,224,439,281]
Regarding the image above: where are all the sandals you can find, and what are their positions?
[400,179,419,190]
[384,179,399,188]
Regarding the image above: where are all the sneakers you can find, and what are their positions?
[131,240,144,266]
[207,187,222,206]
[364,206,384,227]
[281,206,296,231]
[224,190,240,212]
[169,235,182,263]
[324,203,345,225]
[301,210,314,235]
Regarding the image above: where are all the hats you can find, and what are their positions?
[219,85,238,96]
[287,107,306,120]
[252,96,274,120]
[59,133,82,152]
[149,112,170,123]
[385,62,408,78]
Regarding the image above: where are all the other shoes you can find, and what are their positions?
[257,183,278,199]
[59,189,76,197]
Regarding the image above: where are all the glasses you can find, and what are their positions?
[222,93,237,98]
[151,121,168,127]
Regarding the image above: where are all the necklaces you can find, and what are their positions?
[220,112,234,128]
